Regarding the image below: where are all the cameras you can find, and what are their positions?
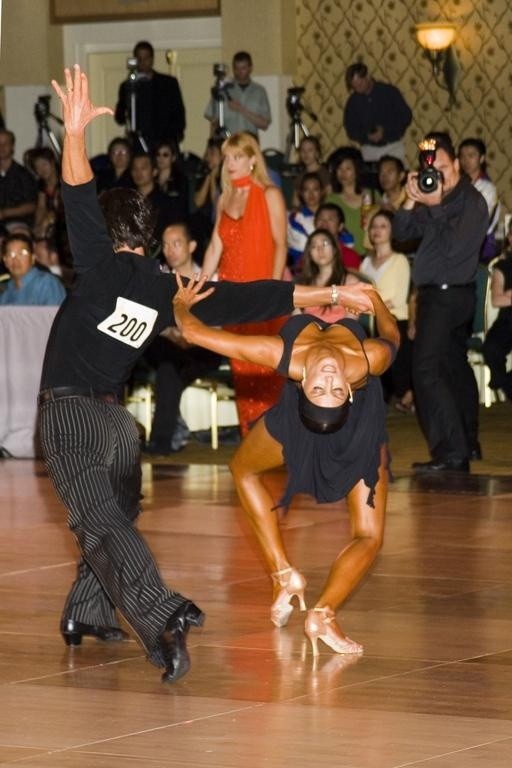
[286,85,320,123]
[33,94,66,126]
[213,61,229,98]
[416,142,446,196]
[128,57,141,80]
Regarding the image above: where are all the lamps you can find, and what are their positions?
[411,21,462,111]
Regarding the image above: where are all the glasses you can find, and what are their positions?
[155,152,169,157]
[111,150,128,155]
[310,240,330,249]
[6,248,30,259]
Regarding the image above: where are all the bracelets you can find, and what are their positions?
[332,284,338,305]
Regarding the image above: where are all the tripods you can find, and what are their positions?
[117,85,151,156]
[212,101,236,142]
[285,117,317,161]
[34,121,64,161]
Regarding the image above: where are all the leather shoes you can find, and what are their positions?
[60,619,129,646]
[469,449,481,461]
[412,461,470,476]
[158,599,205,685]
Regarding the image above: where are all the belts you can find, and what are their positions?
[39,386,119,406]
[417,281,476,292]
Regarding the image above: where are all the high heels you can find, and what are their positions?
[269,567,308,627]
[304,606,364,657]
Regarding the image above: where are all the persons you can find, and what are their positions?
[35,64,375,683]
[322,145,382,256]
[96,137,137,192]
[34,237,74,285]
[188,137,226,211]
[389,130,490,477]
[199,133,294,440]
[283,171,354,266]
[155,140,192,198]
[293,203,361,274]
[457,137,500,350]
[0,232,68,306]
[114,41,185,157]
[357,209,412,411]
[293,136,334,207]
[289,228,360,321]
[29,145,64,240]
[481,217,512,403]
[203,52,272,148]
[148,222,219,457]
[0,129,37,227]
[127,151,172,268]
[378,154,415,211]
[172,272,402,658]
[343,62,412,171]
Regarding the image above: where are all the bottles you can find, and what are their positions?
[360,192,371,230]
